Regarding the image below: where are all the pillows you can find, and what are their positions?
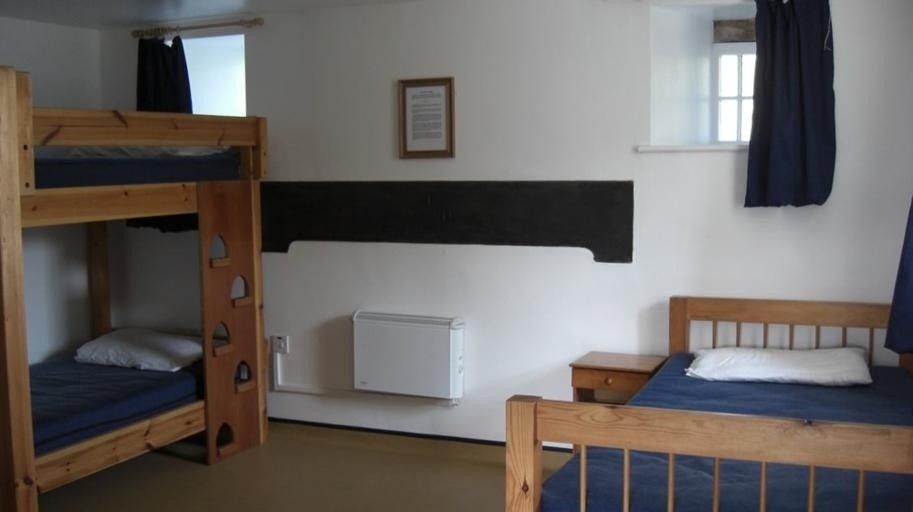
[685,346,873,388]
[74,325,202,374]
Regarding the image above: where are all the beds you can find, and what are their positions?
[2,66,269,510]
[505,294,913,512]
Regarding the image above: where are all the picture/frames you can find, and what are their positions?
[399,75,455,161]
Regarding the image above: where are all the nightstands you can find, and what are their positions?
[567,350,674,454]
